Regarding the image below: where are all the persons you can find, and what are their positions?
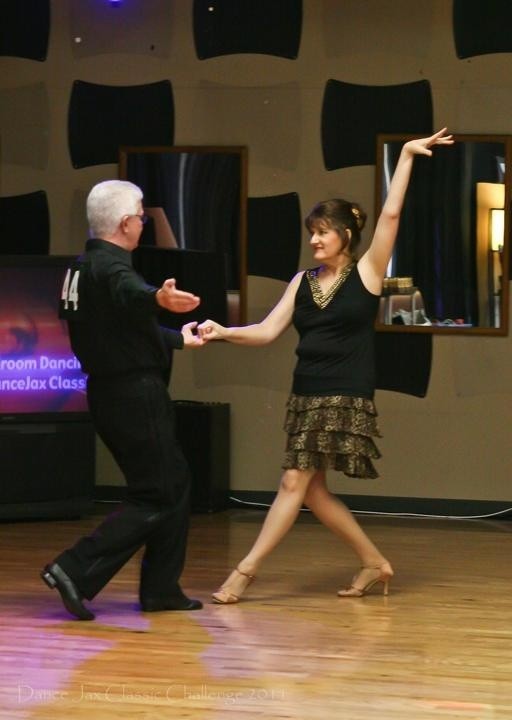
[40,179,213,619]
[0,325,36,362]
[194,126,454,603]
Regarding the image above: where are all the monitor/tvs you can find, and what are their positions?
[0,254,92,424]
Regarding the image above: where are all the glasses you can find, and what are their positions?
[131,214,148,223]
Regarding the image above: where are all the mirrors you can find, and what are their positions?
[372,131,512,334]
[115,142,246,343]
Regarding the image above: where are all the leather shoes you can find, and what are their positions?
[41,560,95,620]
[142,600,203,610]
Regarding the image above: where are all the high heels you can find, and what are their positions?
[212,566,254,603]
[337,560,394,599]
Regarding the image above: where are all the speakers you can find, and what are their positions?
[172,400,230,512]
[133,246,227,341]
